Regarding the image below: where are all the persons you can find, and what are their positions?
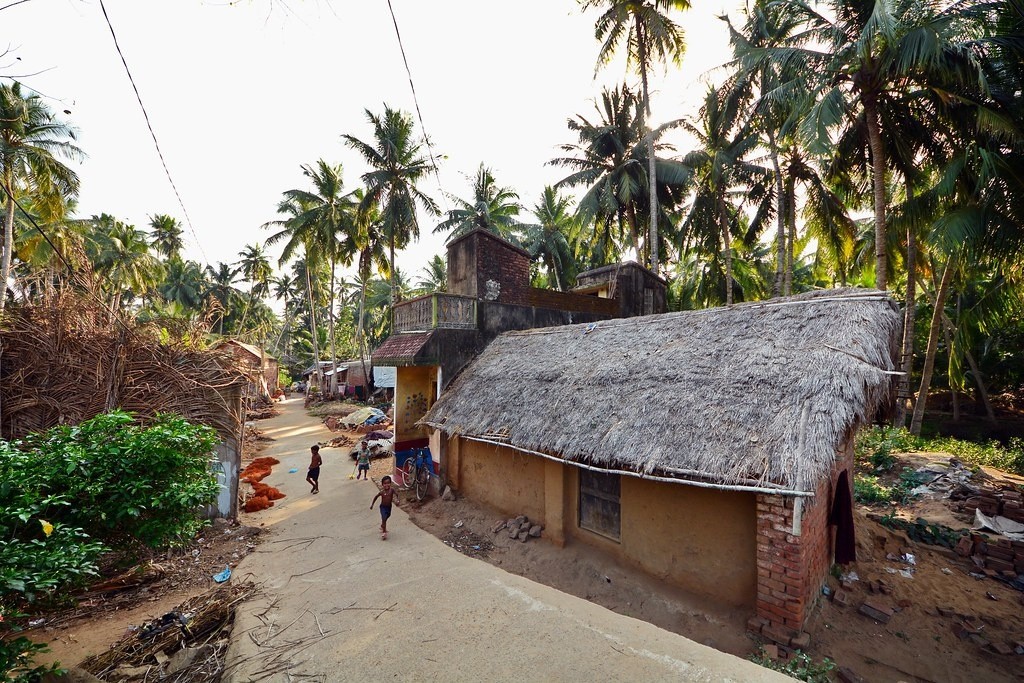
[370,476,400,539]
[356,442,372,480]
[306,445,322,494]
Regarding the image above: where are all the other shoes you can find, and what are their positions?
[382,533,387,540]
[313,490,319,494]
[357,474,361,479]
[364,477,368,480]
[311,488,315,493]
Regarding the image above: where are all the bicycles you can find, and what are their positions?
[402,446,431,501]
